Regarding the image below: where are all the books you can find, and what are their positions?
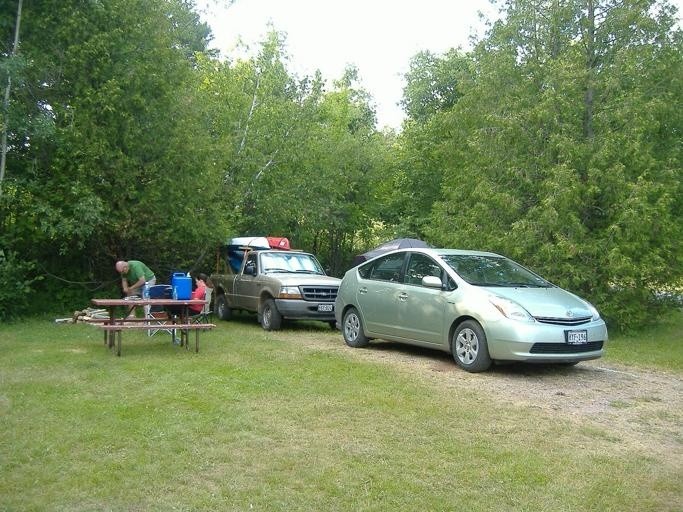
[170,273,192,299]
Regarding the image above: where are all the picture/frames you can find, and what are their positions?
[91,283,209,349]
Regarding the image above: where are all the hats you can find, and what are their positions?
[334,248,608,372]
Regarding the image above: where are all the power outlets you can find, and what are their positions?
[209,250,342,331]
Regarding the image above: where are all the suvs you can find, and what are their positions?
[172,271,193,300]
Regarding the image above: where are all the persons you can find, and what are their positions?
[114,259,156,326]
[163,273,217,325]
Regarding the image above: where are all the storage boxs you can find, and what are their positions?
[89,316,217,357]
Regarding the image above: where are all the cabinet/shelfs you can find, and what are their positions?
[141,281,149,300]
